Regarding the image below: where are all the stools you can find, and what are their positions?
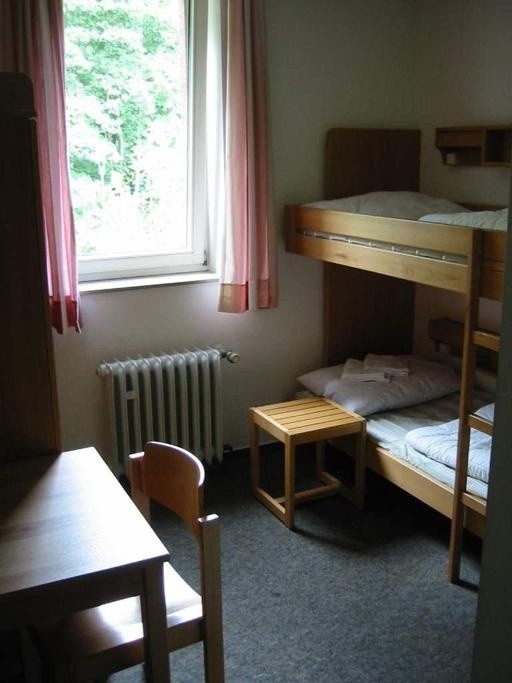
[248,397,367,529]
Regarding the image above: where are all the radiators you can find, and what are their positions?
[95,343,239,475]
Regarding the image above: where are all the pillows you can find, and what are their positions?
[296,354,461,415]
[301,189,470,220]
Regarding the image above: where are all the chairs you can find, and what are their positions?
[25,441,224,683]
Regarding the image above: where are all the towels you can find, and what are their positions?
[341,354,410,383]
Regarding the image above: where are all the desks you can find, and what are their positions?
[0,445,171,683]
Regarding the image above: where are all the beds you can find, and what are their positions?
[282,192,508,581]
